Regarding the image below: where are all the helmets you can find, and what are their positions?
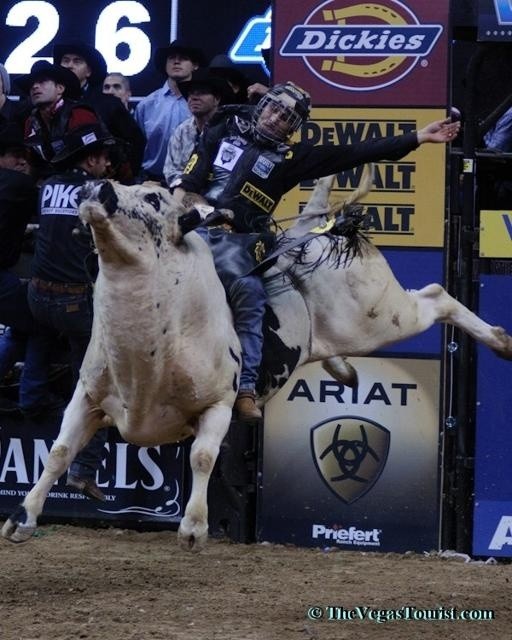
[251,80,313,150]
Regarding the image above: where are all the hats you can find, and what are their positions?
[53,36,107,88]
[49,120,130,166]
[154,38,210,78]
[10,60,82,101]
[176,55,244,107]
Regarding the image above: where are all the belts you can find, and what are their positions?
[30,276,92,297]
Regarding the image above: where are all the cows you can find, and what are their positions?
[0,170,512,545]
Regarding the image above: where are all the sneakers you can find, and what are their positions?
[234,391,263,423]
[66,474,108,504]
[1,391,73,423]
